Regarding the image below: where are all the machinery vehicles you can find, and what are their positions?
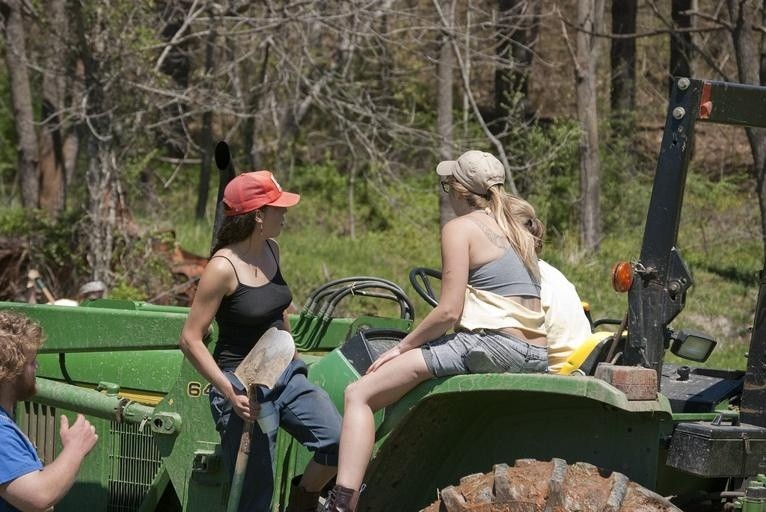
[0,76,765,511]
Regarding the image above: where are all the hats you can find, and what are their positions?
[436,149,506,195]
[222,170,301,216]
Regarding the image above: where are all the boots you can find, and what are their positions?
[285,474,367,512]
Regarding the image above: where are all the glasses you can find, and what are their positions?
[441,180,455,191]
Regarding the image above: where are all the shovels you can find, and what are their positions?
[227,327,295,512]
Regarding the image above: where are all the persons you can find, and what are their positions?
[0,309,99,512]
[179,170,343,512]
[321,150,594,512]
[505,197,593,375]
